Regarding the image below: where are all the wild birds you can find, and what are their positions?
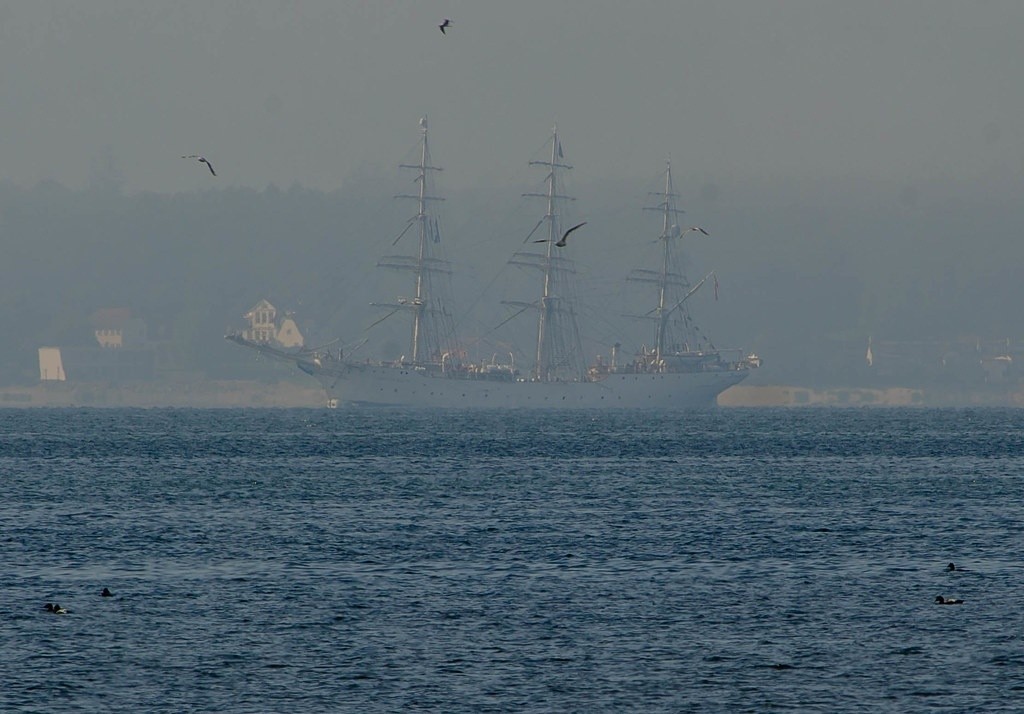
[947,562,971,572]
[98,587,117,597]
[40,602,75,614]
[680,226,709,241]
[530,221,588,248]
[439,19,454,35]
[933,596,966,605]
[181,154,217,177]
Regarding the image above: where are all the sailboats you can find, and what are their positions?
[223,112,761,408]
